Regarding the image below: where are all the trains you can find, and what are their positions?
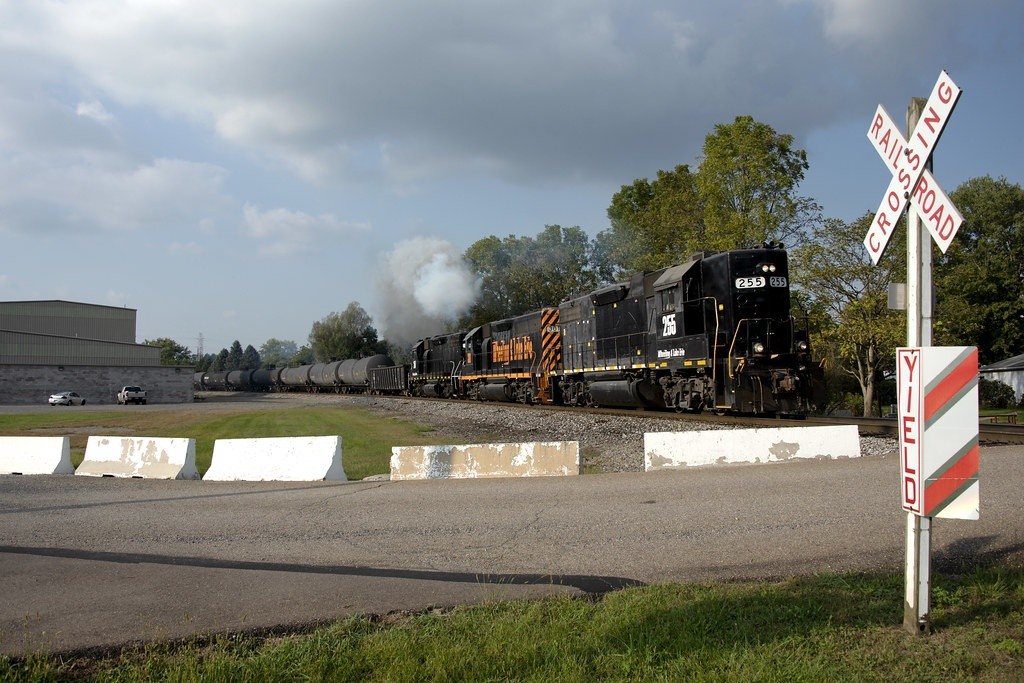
[194,239,817,420]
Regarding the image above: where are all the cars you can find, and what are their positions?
[49,391,86,406]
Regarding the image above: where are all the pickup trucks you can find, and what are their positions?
[116,386,148,405]
